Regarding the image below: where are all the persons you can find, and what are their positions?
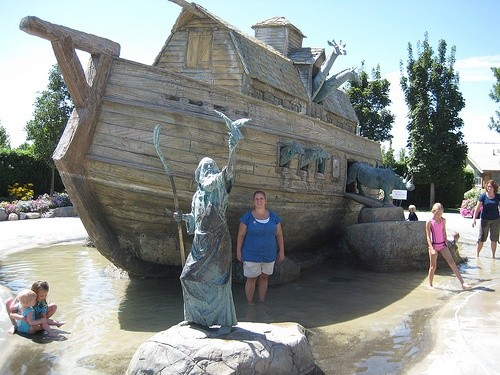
[236,191,284,303]
[407,205,418,221]
[5,280,66,335]
[173,133,238,334]
[425,203,471,288]
[472,180,500,259]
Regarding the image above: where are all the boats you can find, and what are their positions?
[19,0,416,277]
